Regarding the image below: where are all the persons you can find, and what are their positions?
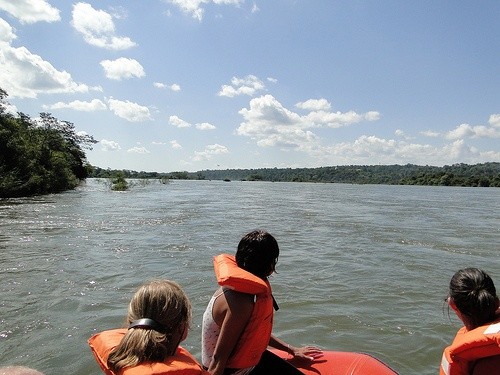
[107,279,191,369]
[199,229,323,375]
[437,268,500,375]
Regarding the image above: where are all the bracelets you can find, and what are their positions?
[287,343,290,356]
